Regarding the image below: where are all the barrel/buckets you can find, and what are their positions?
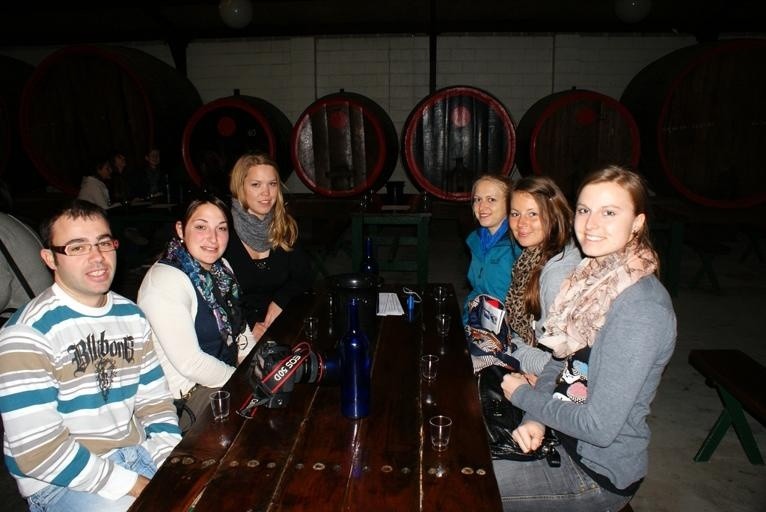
[333,272,383,340]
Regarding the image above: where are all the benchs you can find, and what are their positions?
[302,226,343,281]
[684,241,731,290]
[688,350,766,464]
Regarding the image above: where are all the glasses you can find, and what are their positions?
[46,239,119,256]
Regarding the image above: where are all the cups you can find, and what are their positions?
[429,416,452,452]
[303,318,319,341]
[209,391,230,419]
[421,354,439,383]
[433,287,446,302]
[436,314,451,338]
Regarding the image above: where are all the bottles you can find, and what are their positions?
[361,237,378,280]
[407,289,414,324]
[342,298,371,418]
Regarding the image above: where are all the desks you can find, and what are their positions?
[351,212,430,284]
[111,197,182,248]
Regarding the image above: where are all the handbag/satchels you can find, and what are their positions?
[465,294,512,356]
[476,364,562,468]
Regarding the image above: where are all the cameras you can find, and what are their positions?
[246,341,339,408]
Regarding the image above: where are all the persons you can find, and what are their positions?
[1,143,300,511]
[463,165,678,509]
